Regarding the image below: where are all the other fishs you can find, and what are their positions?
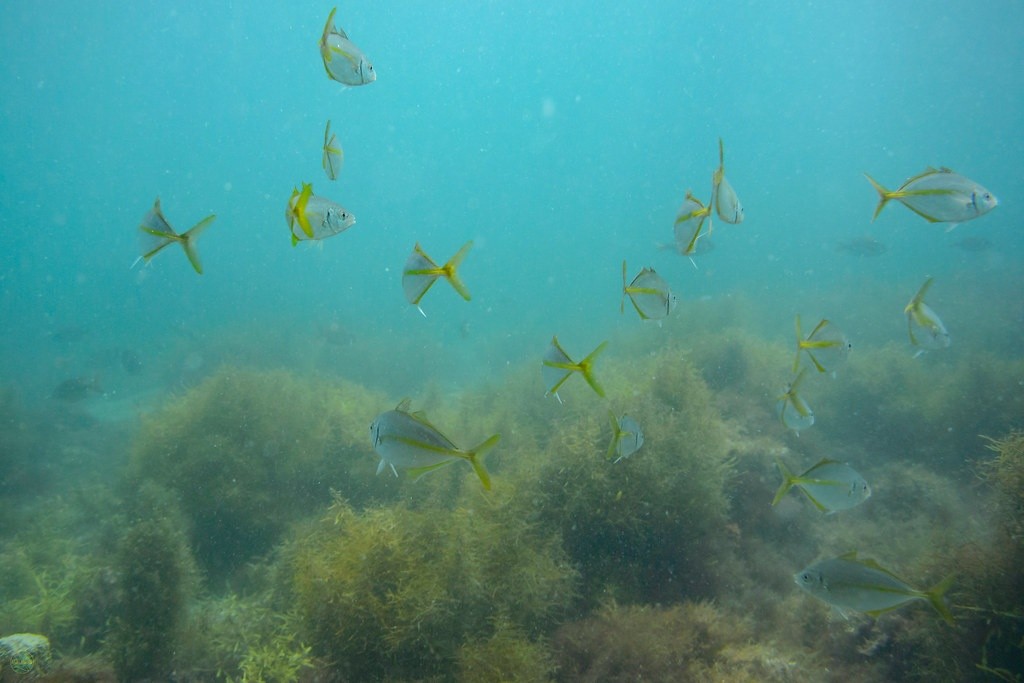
[399,239,473,317]
[863,166,998,231]
[321,118,344,182]
[713,135,745,226]
[673,172,726,257]
[772,458,874,516]
[619,259,677,324]
[319,7,377,88]
[901,274,952,358]
[605,411,646,463]
[137,194,217,274]
[790,548,961,626]
[775,368,817,435]
[368,397,501,494]
[792,317,853,376]
[539,334,612,405]
[284,183,356,246]
